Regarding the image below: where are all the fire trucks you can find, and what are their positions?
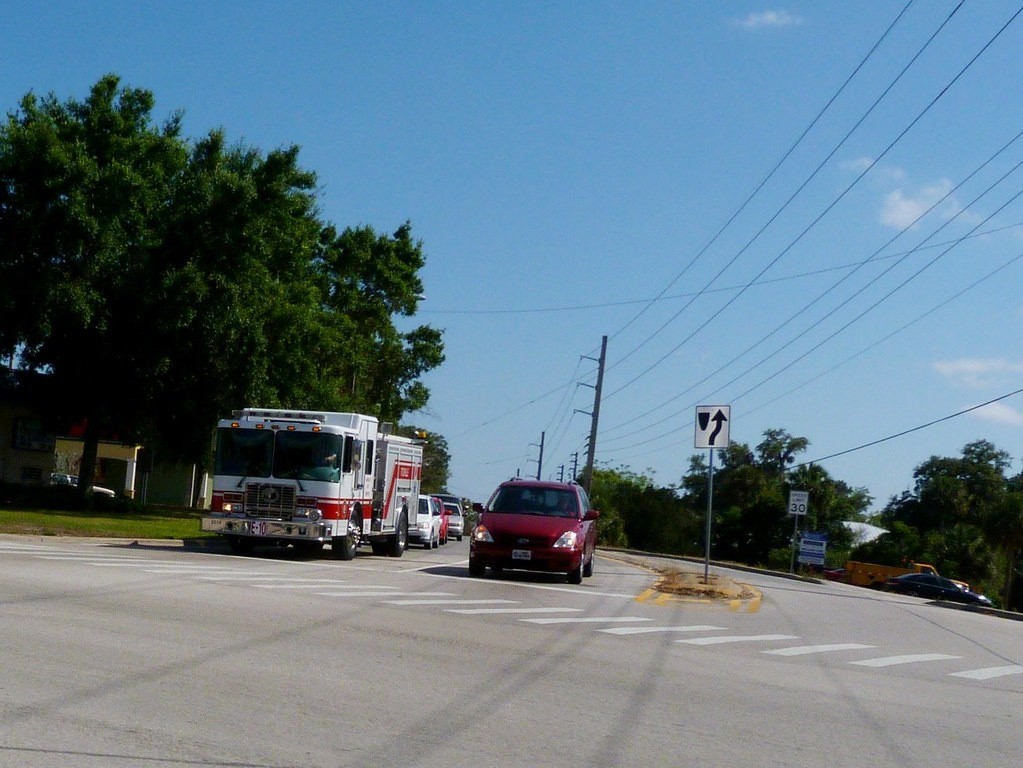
[200,408,427,560]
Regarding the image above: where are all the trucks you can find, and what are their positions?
[847,557,969,595]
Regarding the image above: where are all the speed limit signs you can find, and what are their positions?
[788,490,808,515]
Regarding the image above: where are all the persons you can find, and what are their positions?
[321,448,339,467]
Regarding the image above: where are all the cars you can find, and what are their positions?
[406,495,441,550]
[442,502,468,541]
[434,497,453,544]
[51,473,115,497]
[466,477,601,583]
[825,568,847,583]
[890,573,983,606]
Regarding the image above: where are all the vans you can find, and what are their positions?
[431,495,466,515]
